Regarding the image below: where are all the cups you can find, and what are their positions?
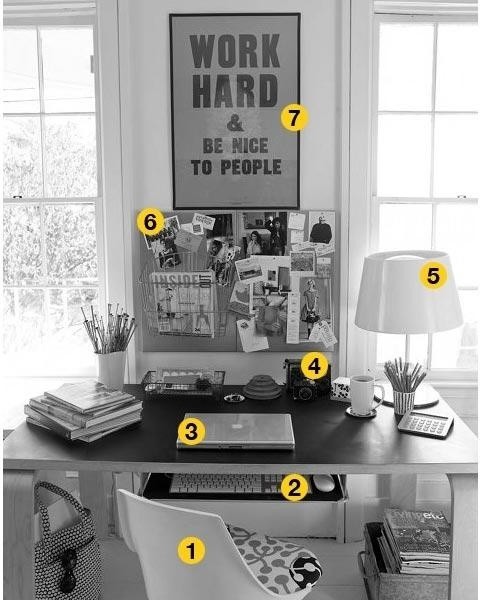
[393,388,415,416]
[349,376,385,415]
[98,351,126,390]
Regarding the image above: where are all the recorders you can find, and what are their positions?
[286,359,331,401]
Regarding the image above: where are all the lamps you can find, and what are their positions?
[353,245,461,377]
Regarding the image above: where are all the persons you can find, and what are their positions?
[300,279,321,340]
[310,215,333,244]
[160,237,181,267]
[206,214,286,288]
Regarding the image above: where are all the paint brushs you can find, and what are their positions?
[81,303,138,354]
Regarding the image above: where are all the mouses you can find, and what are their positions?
[313,474,336,493]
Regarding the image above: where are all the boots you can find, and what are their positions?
[307,328,311,340]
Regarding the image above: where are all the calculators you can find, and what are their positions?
[398,409,454,439]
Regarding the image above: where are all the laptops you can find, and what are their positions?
[176,413,295,450]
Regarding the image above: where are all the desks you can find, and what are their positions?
[3,377,480,599]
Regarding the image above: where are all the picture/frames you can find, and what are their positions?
[166,13,304,212]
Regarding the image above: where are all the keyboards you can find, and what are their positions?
[170,473,313,494]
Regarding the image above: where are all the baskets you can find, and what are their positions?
[136,253,235,337]
[139,369,225,401]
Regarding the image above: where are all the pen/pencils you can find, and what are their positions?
[383,357,427,393]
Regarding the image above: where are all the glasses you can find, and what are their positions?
[59,549,77,594]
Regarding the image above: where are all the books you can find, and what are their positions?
[23,376,145,444]
[376,508,449,577]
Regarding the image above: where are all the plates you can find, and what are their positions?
[345,406,378,417]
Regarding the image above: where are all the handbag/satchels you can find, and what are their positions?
[306,311,320,323]
[34,480,105,599]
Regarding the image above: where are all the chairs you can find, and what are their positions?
[115,486,321,598]
